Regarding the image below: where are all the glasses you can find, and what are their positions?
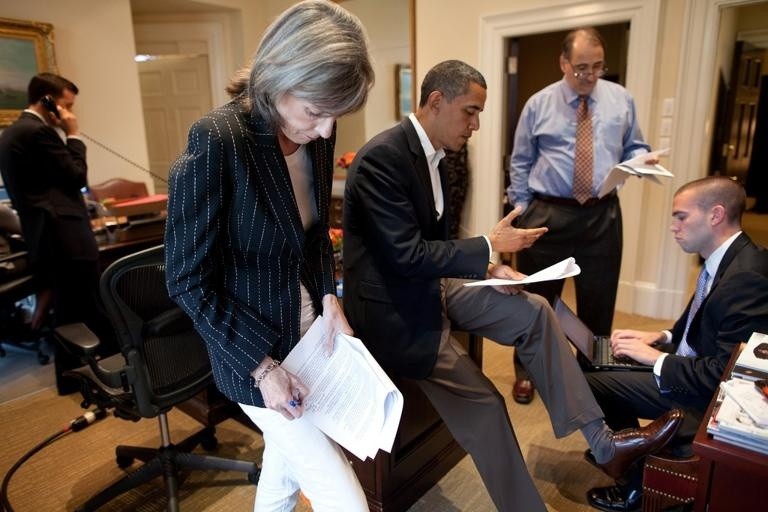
[566,56,608,79]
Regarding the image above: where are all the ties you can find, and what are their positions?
[678,264,709,357]
[573,95,593,204]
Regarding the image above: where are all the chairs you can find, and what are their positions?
[0,208,48,366]
[53,243,260,512]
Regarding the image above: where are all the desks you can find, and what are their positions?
[640,340,768,511]
[334,265,484,512]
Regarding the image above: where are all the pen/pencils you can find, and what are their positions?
[289,399,302,406]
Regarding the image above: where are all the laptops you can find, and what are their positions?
[552,295,668,373]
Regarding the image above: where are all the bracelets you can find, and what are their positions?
[254,360,281,388]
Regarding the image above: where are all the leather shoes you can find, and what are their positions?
[513,376,535,404]
[587,486,643,512]
[596,408,685,486]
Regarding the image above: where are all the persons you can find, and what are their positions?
[166,1,374,512]
[508,26,660,404]
[0,72,112,396]
[343,61,684,512]
[585,176,768,512]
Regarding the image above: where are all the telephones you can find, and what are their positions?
[40,94,60,119]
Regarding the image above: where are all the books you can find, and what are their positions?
[707,331,768,457]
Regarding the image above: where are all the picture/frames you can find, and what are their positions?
[0,15,61,125]
[394,63,411,121]
